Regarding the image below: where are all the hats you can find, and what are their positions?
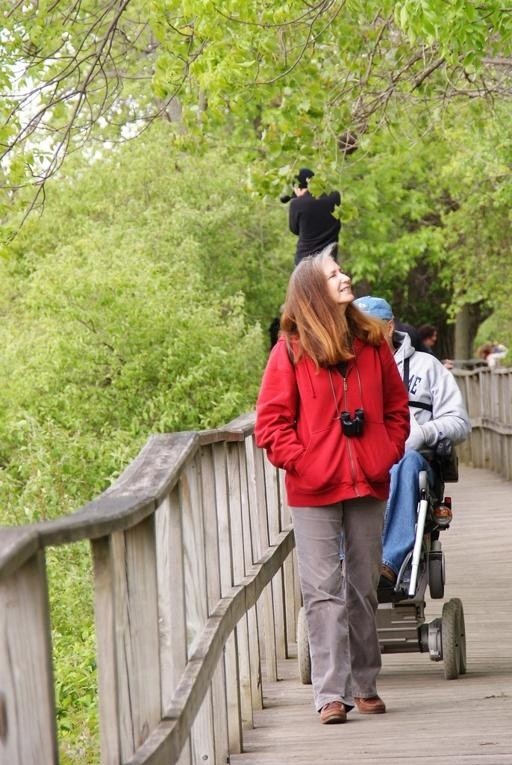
[351,296,392,320]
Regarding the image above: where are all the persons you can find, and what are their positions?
[413,321,454,371]
[268,302,286,349]
[254,246,412,729]
[289,165,341,271]
[353,292,474,603]
[478,338,508,372]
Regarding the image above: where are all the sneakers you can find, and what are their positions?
[378,564,395,587]
[354,695,385,714]
[320,701,346,724]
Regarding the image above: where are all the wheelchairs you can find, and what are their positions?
[290,436,466,686]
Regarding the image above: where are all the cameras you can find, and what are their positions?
[279,190,296,203]
